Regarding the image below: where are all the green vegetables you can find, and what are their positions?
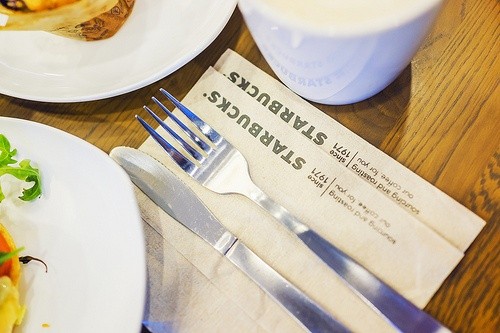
[0,134,42,201]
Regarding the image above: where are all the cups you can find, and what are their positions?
[237,1,442,107]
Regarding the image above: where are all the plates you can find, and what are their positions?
[0,116,149,332]
[0,1,235,104]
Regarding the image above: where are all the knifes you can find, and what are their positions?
[107,142,350,333]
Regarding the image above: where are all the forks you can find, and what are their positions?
[132,85,449,333]
